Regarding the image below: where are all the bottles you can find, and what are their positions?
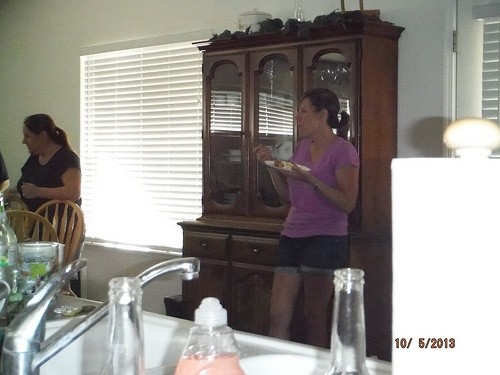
[101,278,144,375]
[0,191,17,293]
[294,0,305,21]
[323,268,370,375]
[173,297,247,375]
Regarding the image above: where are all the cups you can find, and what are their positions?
[17,240,59,286]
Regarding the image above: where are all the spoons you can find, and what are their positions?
[268,143,284,149]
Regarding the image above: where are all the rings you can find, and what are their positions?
[257,153,258,157]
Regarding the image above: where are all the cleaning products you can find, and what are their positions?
[175,298,245,374]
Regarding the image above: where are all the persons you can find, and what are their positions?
[3,113,85,298]
[251,88,359,347]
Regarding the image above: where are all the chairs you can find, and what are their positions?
[4,193,86,297]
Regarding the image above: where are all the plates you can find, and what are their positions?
[264,161,312,175]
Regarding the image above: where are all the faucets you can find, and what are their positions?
[0,254,201,375]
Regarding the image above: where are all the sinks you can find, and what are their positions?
[42,313,248,374]
[149,349,326,375]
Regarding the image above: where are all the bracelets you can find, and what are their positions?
[312,181,319,191]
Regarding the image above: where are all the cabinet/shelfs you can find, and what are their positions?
[175,20,406,360]
